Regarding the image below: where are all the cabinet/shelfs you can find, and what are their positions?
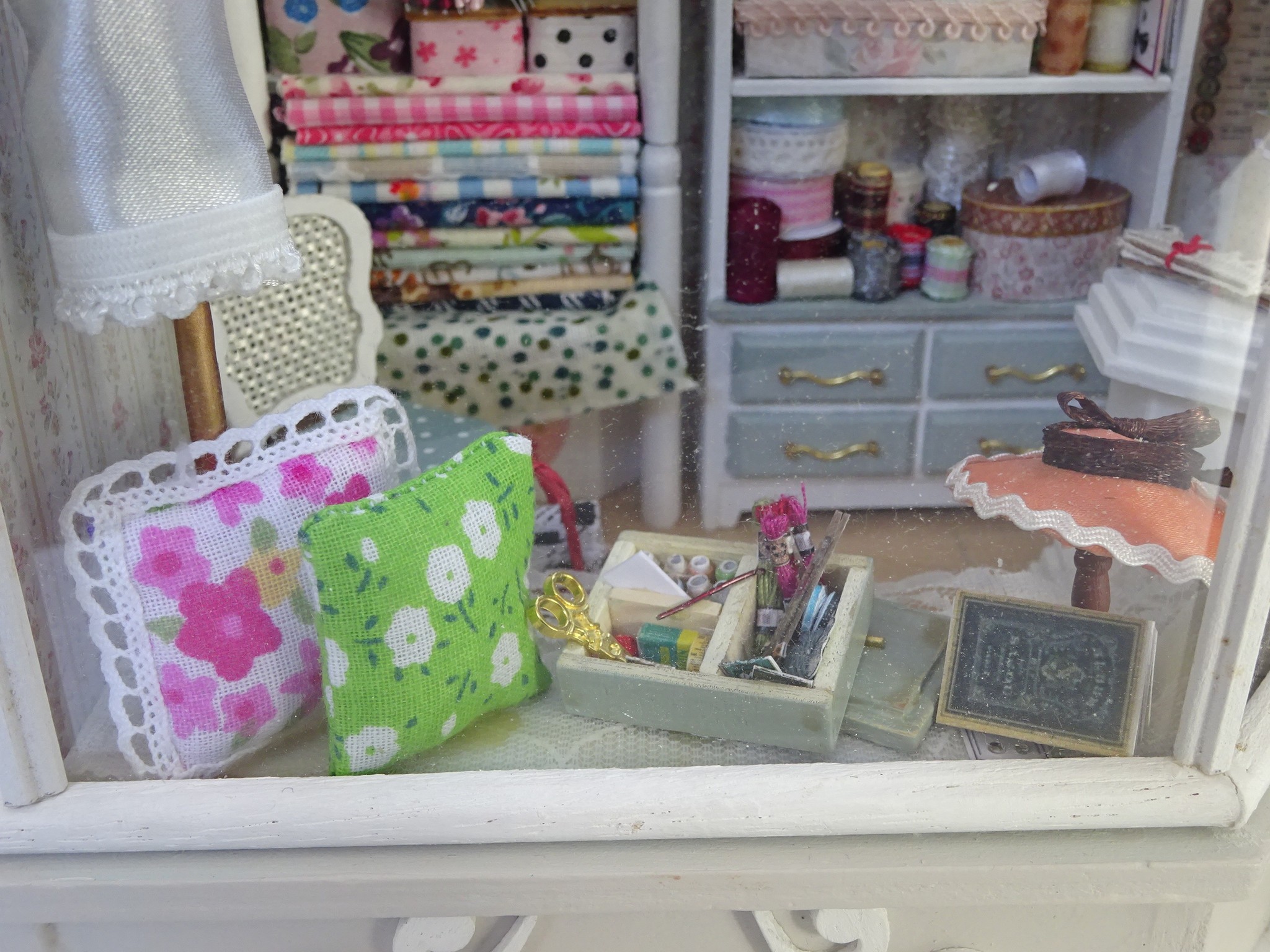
[705,0,1211,527]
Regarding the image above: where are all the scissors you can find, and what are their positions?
[527,572,640,675]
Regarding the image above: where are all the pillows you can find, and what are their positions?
[58,386,425,777]
[298,425,552,775]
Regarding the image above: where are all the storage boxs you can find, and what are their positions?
[524,0,637,76]
[736,4,1049,77]
[958,178,1133,302]
[407,8,527,80]
[557,526,878,753]
